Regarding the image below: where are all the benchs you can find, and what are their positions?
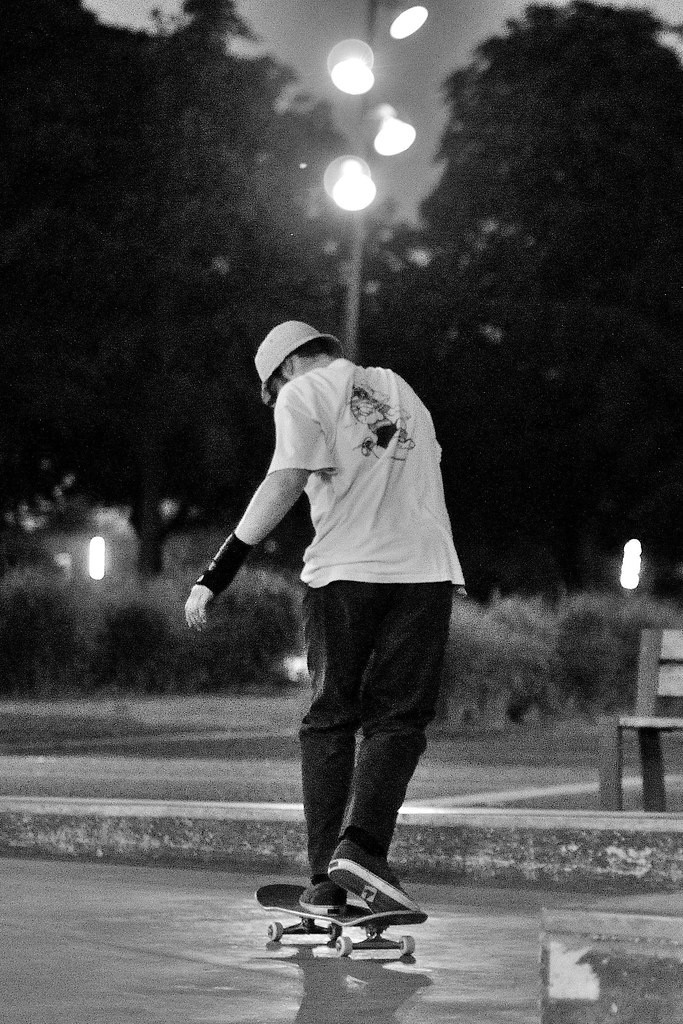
[598,628,683,815]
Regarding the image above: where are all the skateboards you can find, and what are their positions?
[254,884,428,957]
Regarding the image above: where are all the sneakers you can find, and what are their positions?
[327,839,419,915]
[299,881,348,917]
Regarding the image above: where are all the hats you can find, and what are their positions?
[254,320,344,409]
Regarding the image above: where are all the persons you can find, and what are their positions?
[186,321,467,915]
